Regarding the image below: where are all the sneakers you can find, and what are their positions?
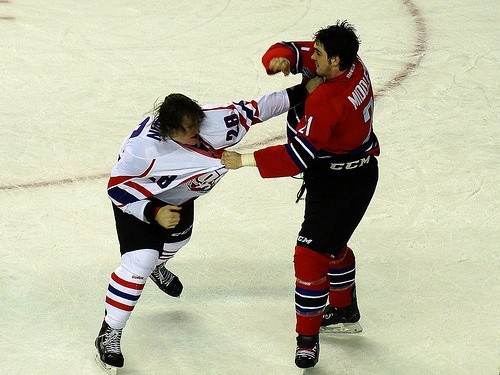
[94,318,125,375]
[149,262,183,299]
[295,332,320,375]
[318,300,363,334]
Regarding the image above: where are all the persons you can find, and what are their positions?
[220,20,380,375]
[94,77,325,375]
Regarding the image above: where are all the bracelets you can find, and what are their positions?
[241,153,257,167]
